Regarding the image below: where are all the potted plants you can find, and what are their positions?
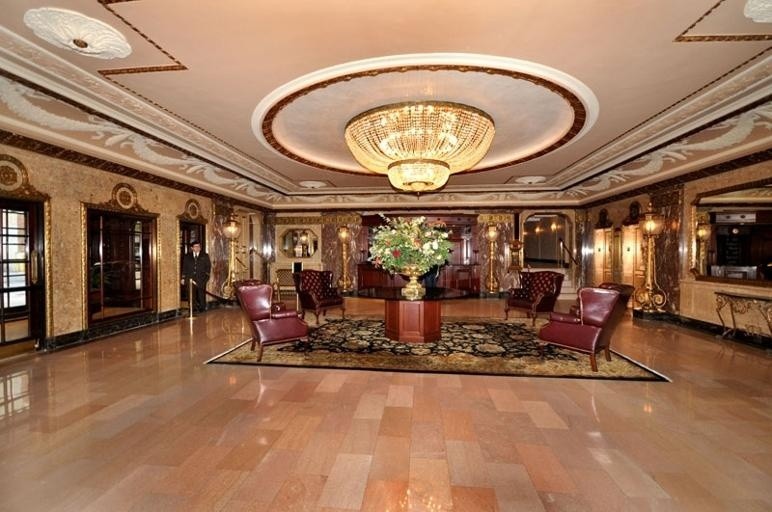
[88,267,110,305]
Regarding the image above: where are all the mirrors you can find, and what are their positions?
[688,177,772,282]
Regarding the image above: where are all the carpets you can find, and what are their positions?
[204,310,676,382]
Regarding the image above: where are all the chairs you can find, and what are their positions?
[233,269,345,362]
[504,271,637,372]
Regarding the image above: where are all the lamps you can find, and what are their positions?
[335,226,354,244]
[637,211,665,238]
[485,225,500,243]
[695,223,713,242]
[344,102,495,198]
[223,218,240,241]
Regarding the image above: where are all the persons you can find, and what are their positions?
[425,259,450,287]
[180,241,210,312]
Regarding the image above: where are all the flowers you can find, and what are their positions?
[368,212,453,277]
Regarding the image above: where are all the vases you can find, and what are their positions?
[400,267,426,302]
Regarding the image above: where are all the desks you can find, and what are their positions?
[713,289,772,347]
[385,291,443,342]
